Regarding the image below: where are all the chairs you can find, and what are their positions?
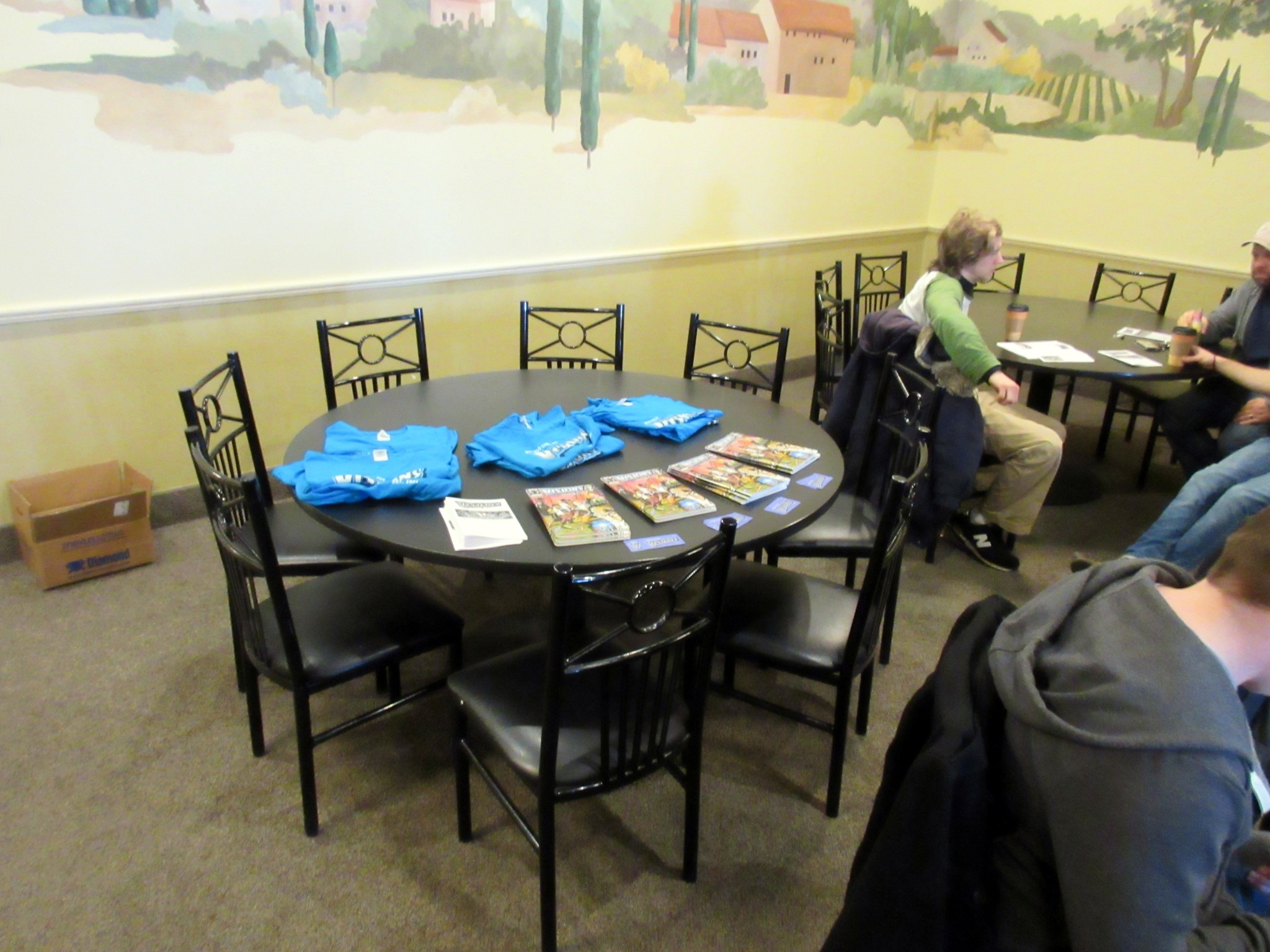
[1096,286,1239,472]
[1060,260,1177,441]
[441,513,739,952]
[752,350,951,666]
[973,251,1029,296]
[681,309,791,406]
[807,299,853,425]
[175,347,396,699]
[811,259,849,381]
[852,248,910,342]
[181,423,466,840]
[712,425,935,805]
[317,304,432,412]
[517,295,627,371]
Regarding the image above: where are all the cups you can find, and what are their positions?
[1004,303,1030,342]
[1168,326,1197,367]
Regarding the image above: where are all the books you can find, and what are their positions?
[435,433,818,554]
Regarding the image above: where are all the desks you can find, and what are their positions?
[280,366,850,631]
[967,291,1210,509]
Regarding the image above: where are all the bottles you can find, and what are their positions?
[1187,308,1204,356]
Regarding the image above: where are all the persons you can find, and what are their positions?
[989,503,1270,952]
[1160,221,1269,489]
[1119,431,1270,587]
[894,210,1071,570]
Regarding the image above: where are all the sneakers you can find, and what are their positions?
[953,510,1020,572]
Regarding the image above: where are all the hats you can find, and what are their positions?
[1242,222,1270,252]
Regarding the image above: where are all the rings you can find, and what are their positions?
[1249,414,1253,418]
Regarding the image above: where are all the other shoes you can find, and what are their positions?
[1069,556,1095,571]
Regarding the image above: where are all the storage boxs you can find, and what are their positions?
[5,457,161,589]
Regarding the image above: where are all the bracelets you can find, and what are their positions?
[1211,354,1218,371]
